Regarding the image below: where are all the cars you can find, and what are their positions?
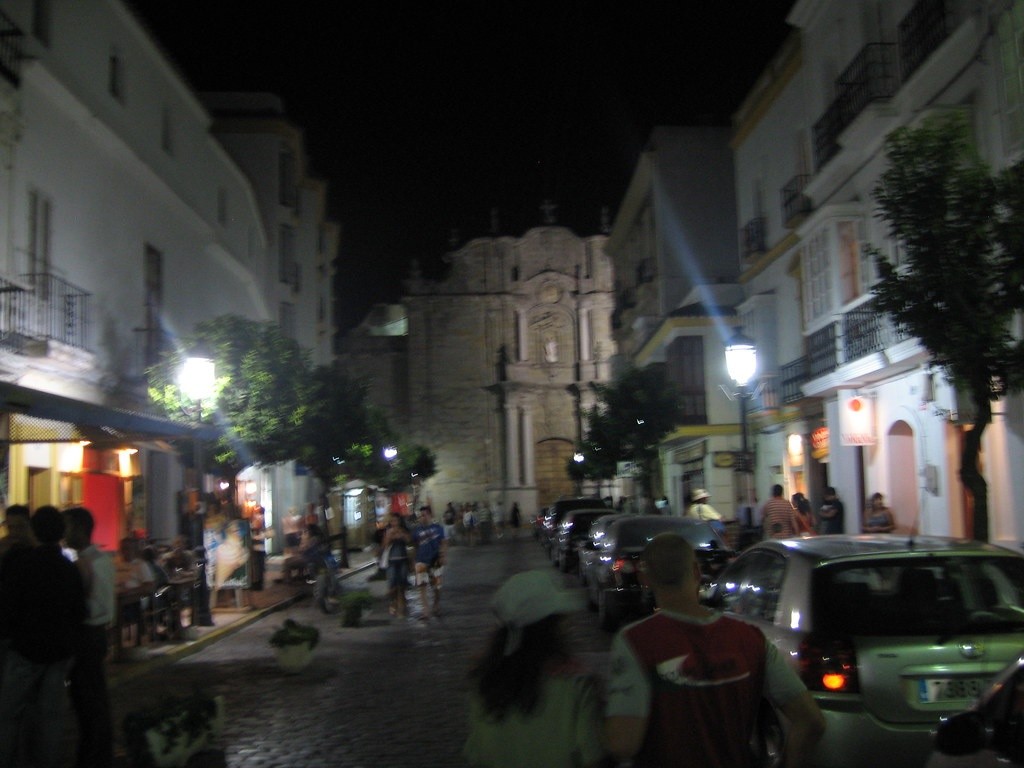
[701,533,1023,768]
[926,651,1024,768]
[528,499,729,632]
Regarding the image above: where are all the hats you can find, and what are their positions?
[491,571,580,656]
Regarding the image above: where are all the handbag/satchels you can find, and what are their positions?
[379,544,393,568]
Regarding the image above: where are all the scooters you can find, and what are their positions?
[312,543,344,615]
[689,539,741,593]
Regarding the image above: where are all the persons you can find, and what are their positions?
[282,495,329,585]
[601,533,827,768]
[819,488,844,533]
[614,493,672,515]
[443,501,504,547]
[375,505,445,620]
[860,494,897,533]
[0,504,118,768]
[462,567,605,768]
[510,502,521,537]
[106,536,195,662]
[686,490,721,525]
[758,484,817,540]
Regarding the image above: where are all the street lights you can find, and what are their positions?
[177,338,212,626]
[721,324,758,549]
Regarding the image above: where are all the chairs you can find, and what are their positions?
[114,573,200,663]
[875,567,941,623]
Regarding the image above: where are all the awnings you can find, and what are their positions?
[0,381,221,443]
